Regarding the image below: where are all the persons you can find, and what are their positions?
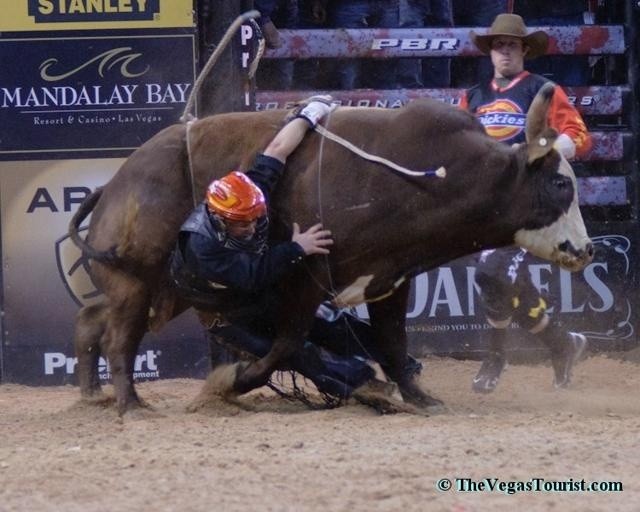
[167,94,424,405]
[457,35,593,396]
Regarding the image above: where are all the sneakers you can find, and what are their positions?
[472,348,513,397]
[549,327,593,392]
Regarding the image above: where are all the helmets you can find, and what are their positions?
[202,170,271,256]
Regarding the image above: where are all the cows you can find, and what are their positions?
[69,82,596,418]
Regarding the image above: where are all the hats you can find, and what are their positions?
[467,13,551,60]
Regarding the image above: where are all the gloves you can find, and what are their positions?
[296,94,338,127]
